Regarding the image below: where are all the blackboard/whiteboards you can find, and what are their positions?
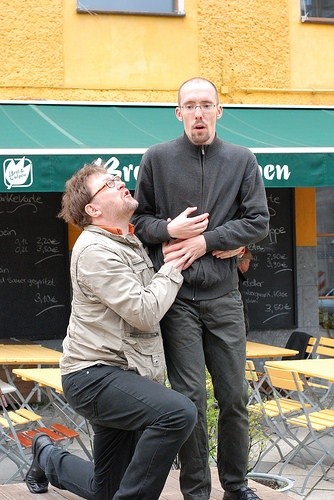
[0,185,302,344]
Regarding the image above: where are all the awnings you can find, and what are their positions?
[0,104,334,192]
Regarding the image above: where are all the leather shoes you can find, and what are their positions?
[223,486,263,500]
[25,433,53,494]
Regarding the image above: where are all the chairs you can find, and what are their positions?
[0,377,79,484]
[244,331,334,494]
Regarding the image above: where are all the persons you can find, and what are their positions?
[25,163,246,500]
[129,77,270,500]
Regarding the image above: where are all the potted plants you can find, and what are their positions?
[205,366,294,495]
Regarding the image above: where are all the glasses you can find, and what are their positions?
[86,176,121,205]
[180,101,218,112]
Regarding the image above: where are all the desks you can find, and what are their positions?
[0,345,94,463]
[246,341,334,466]
